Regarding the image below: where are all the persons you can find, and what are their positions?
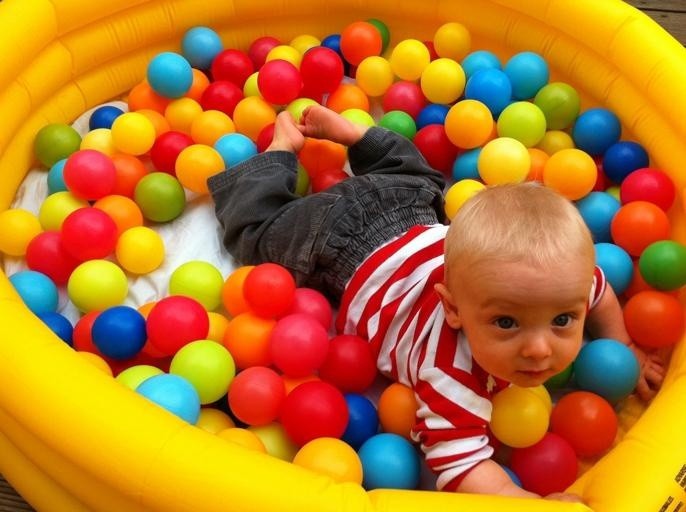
[197,99,667,503]
